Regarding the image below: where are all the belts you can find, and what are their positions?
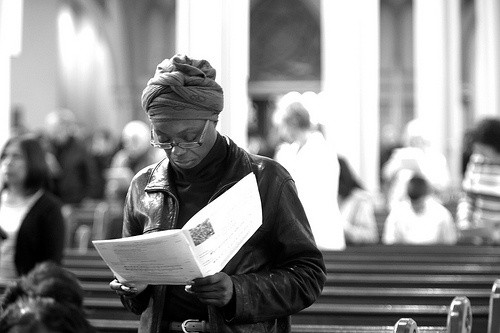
[168,318,209,333]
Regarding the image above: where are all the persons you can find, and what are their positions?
[108,54,327,333]
[270,100,500,252]
[38,110,170,207]
[0,259,90,333]
[0,138,65,296]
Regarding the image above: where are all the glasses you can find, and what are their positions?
[148,121,210,149]
